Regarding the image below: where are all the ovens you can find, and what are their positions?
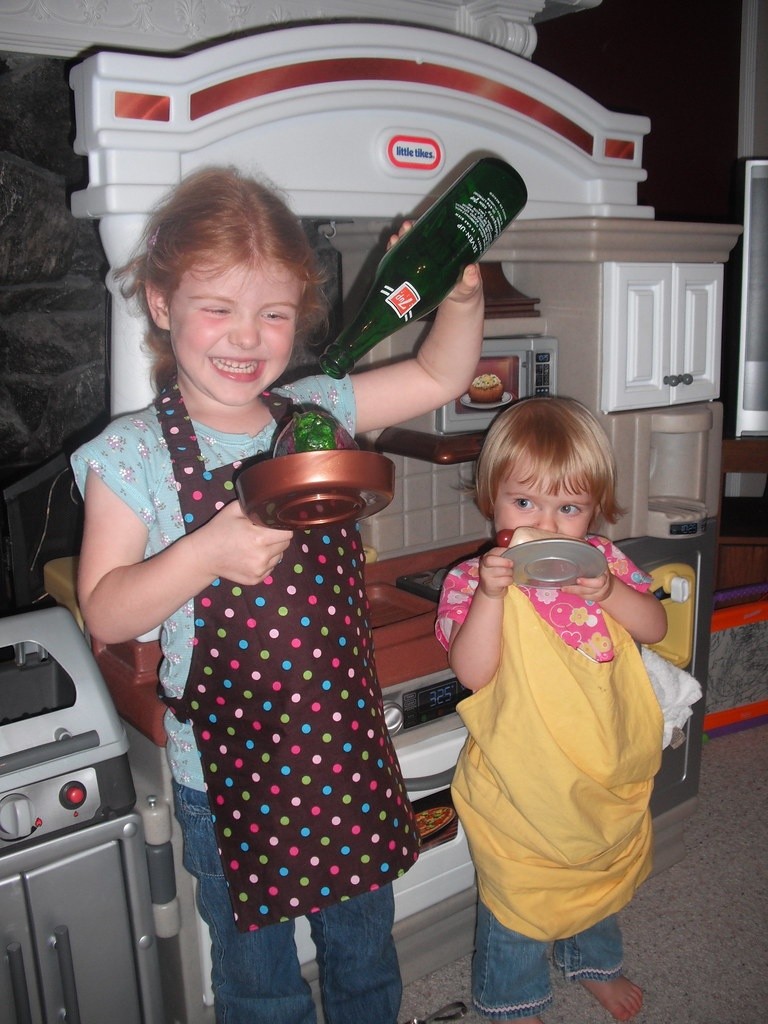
[200,669,476,1007]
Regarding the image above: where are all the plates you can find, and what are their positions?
[499,538,606,590]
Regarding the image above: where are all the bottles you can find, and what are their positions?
[318,157,527,380]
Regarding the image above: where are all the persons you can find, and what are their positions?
[71,167,486,1024]
[434,394,668,1024]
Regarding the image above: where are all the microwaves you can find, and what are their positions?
[386,338,559,437]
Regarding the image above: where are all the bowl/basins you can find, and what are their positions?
[235,448,396,530]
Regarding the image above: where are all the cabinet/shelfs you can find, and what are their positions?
[67,18,768,1024]
